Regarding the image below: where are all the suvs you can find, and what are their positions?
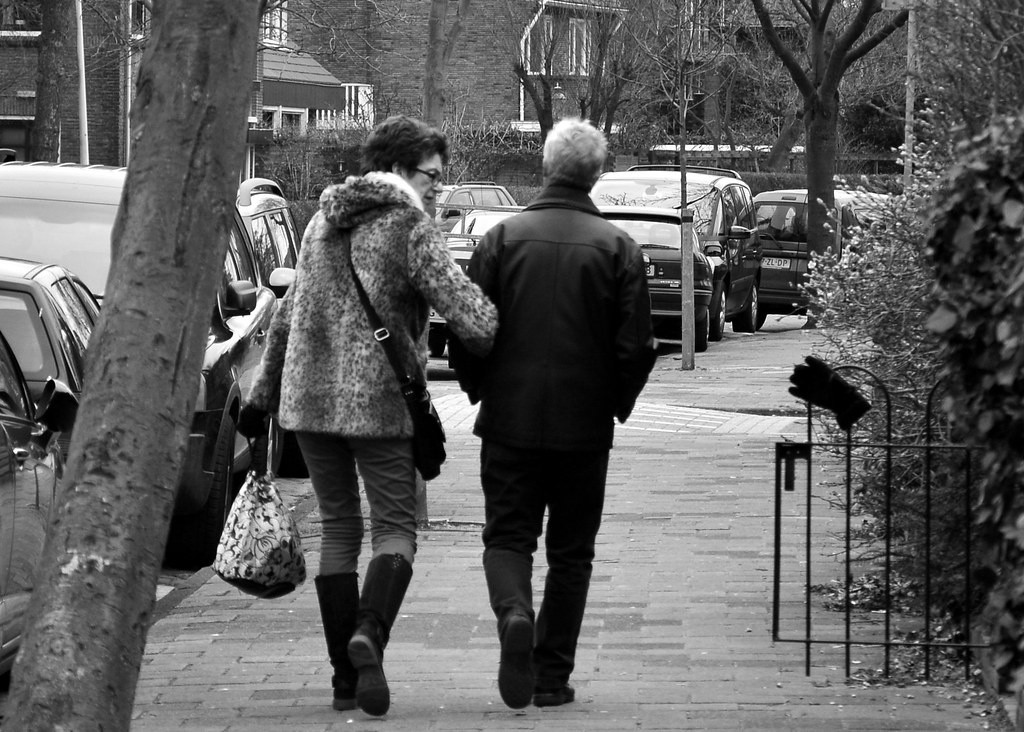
[589,170,764,341]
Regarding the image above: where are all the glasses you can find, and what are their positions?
[414,168,442,187]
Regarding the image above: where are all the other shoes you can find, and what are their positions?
[533,681,575,706]
[497,614,535,709]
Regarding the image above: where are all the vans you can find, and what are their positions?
[740,187,860,329]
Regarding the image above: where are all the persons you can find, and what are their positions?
[237,112,500,715]
[446,117,656,710]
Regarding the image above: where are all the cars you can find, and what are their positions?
[596,205,724,354]
[0,161,532,677]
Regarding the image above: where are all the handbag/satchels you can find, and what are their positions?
[210,434,306,600]
[409,388,448,481]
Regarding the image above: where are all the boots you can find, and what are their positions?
[313,572,361,710]
[346,551,412,715]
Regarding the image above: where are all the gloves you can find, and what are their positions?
[234,404,269,437]
[788,355,873,431]
